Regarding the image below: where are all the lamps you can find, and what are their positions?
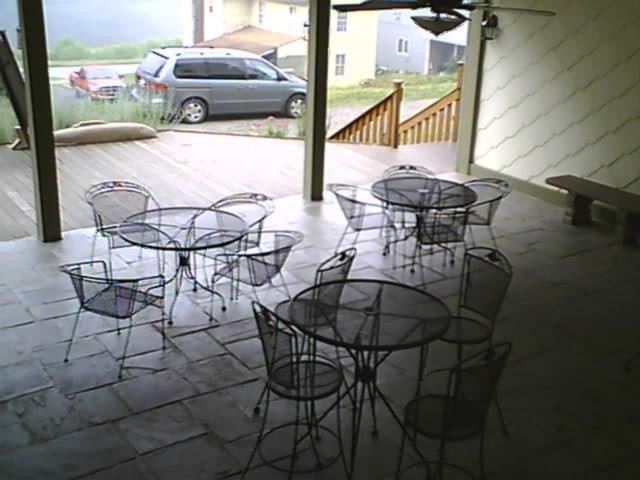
[412,13,466,36]
[480,14,500,40]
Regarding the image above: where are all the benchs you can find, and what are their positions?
[545,175,639,245]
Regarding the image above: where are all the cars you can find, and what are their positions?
[130,47,309,123]
[69,65,128,103]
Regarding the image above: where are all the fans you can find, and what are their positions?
[331,0,557,37]
